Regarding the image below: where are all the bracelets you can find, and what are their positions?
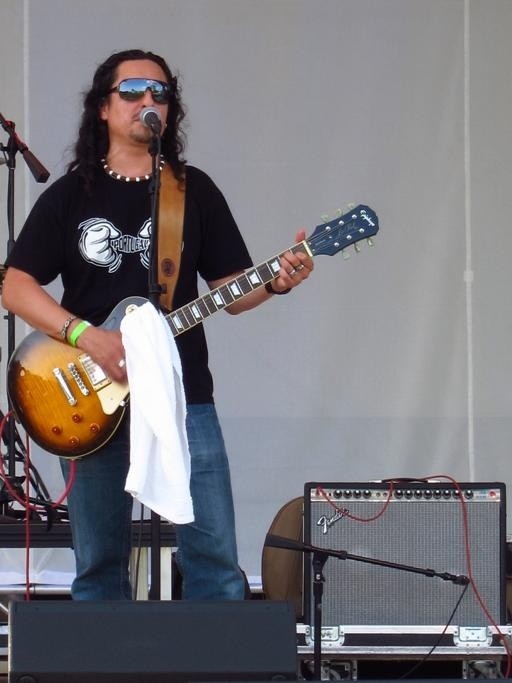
[69,320,92,347]
[60,315,80,344]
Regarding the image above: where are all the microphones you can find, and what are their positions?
[265,534,347,561]
[139,106,161,133]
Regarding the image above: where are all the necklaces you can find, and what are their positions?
[101,152,165,183]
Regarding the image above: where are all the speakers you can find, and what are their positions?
[7,599,298,683]
[303,481,506,646]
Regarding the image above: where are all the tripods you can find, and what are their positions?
[0,137,68,523]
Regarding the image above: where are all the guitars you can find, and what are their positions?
[8,204,379,460]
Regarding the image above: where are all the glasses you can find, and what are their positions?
[108,78,172,104]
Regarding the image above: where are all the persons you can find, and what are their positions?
[0,49,316,602]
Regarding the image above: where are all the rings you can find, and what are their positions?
[117,359,126,368]
[295,264,304,271]
[289,269,297,277]
